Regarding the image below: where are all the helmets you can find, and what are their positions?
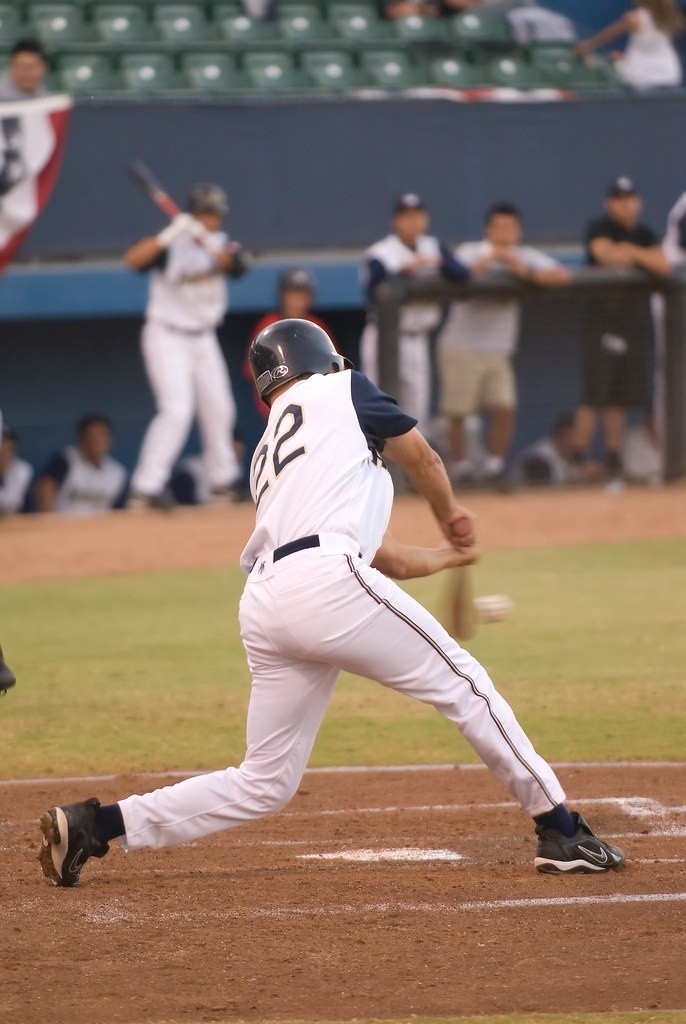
[249,318,353,409]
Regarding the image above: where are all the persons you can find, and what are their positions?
[38,319,631,886]
[358,194,472,429]
[502,415,598,491]
[178,434,244,506]
[578,0,686,92]
[0,39,51,101]
[573,175,671,482]
[651,192,686,450]
[0,429,39,513]
[244,269,332,420]
[436,202,574,484]
[124,183,252,509]
[35,414,128,513]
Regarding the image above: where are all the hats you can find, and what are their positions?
[606,175,636,197]
[193,184,229,213]
[275,267,315,291]
[393,193,424,213]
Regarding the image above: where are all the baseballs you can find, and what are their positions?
[472,594,513,624]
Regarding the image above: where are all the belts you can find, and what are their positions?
[248,534,363,573]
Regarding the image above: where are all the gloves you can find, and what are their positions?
[158,213,191,244]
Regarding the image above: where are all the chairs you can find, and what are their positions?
[0,0,619,96]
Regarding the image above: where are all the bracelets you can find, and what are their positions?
[525,268,533,279]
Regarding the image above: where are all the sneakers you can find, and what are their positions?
[534,811,624,875]
[39,797,109,886]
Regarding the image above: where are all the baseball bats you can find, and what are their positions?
[451,518,481,641]
[128,159,205,246]
